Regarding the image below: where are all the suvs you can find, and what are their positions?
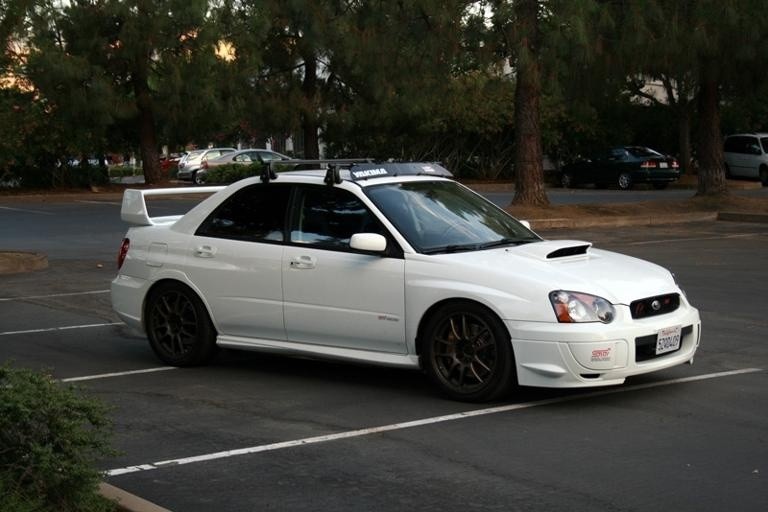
[720,133,767,188]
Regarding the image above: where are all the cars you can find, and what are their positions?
[197,149,310,182]
[177,148,238,186]
[557,147,681,189]
[110,160,703,404]
